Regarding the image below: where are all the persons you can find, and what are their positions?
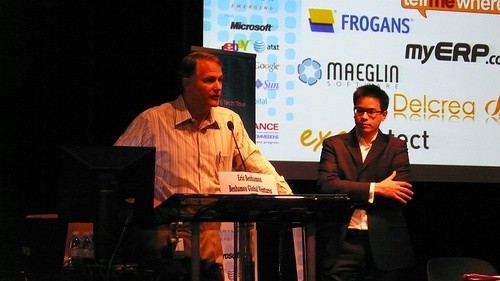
[113,52,294,281]
[315,84,414,281]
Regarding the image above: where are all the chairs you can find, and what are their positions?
[426,256,497,281]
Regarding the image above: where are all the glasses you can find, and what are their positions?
[353,106,384,117]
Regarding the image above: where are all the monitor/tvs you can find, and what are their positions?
[54,145,156,269]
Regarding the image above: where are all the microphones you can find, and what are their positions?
[227,121,247,172]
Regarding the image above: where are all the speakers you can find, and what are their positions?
[191,45,257,146]
